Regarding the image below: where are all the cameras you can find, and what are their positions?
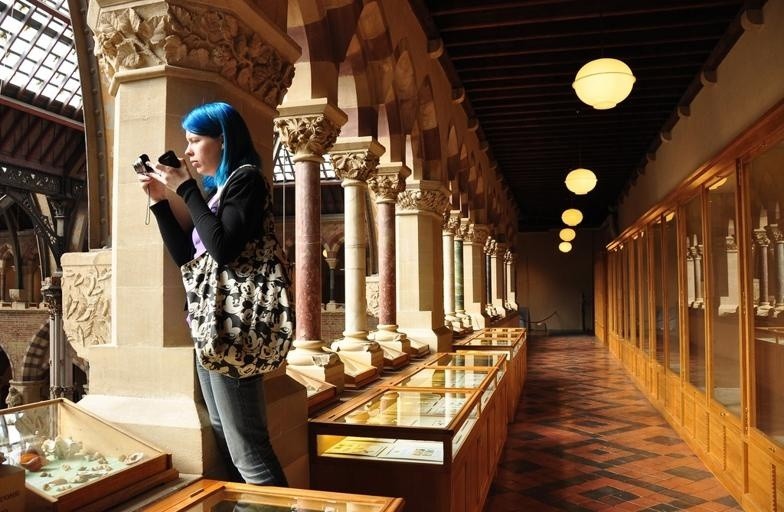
[132,154,153,174]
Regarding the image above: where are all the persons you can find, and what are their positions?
[138,100,290,486]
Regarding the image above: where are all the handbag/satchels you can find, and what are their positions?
[179,234,294,379]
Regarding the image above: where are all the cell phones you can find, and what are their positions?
[158,150,181,168]
